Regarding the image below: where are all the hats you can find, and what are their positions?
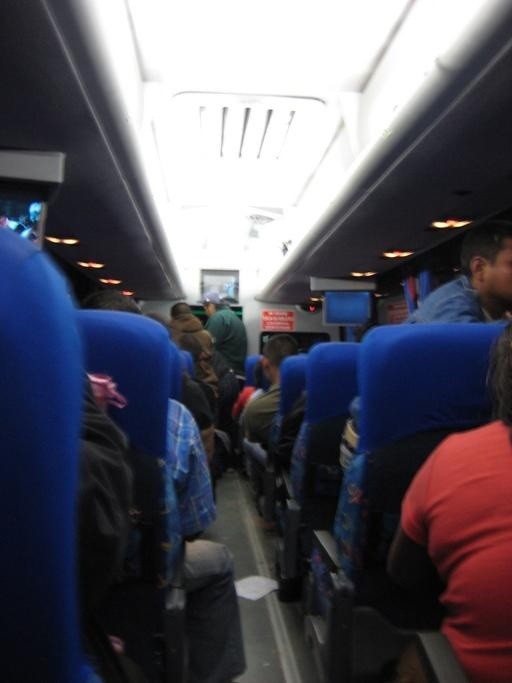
[196,290,221,305]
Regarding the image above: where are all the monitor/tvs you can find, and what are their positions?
[201,270,239,304]
[323,289,373,328]
[0,200,46,250]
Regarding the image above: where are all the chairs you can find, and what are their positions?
[1,220,201,680]
[240,318,507,679]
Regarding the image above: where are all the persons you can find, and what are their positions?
[83,288,247,682]
[167,288,247,459]
[232,333,299,450]
[336,226,512,473]
[77,370,141,682]
[384,319,510,681]
